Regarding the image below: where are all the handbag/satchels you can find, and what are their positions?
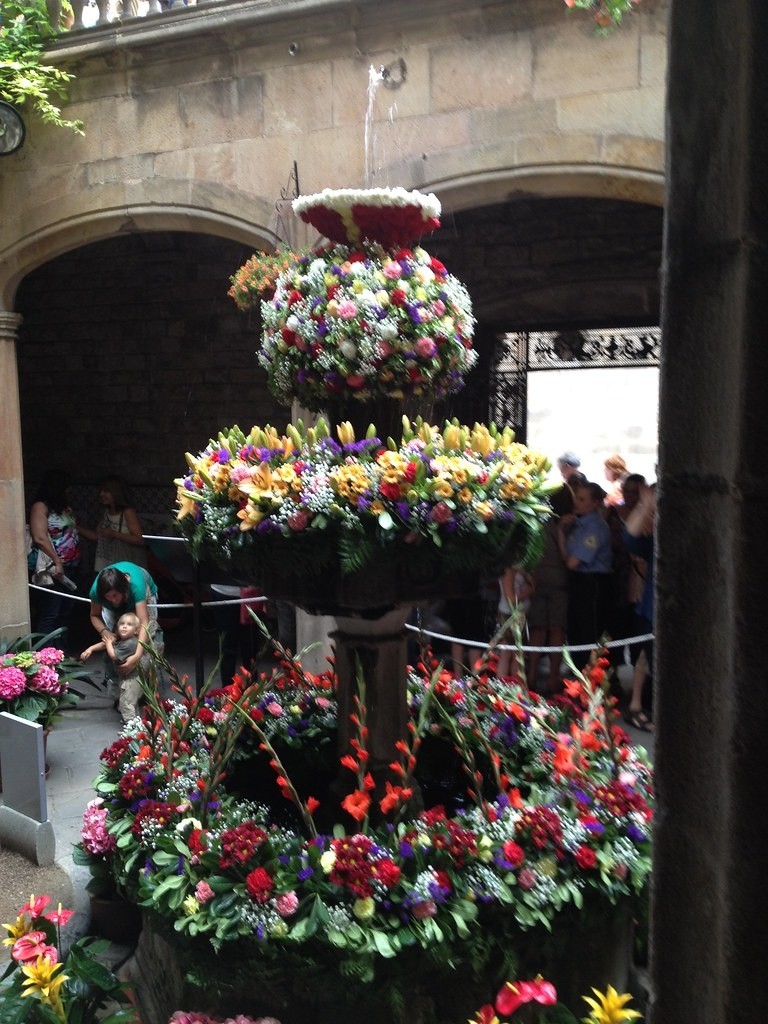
[110,511,144,562]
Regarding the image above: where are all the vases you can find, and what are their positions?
[42,722,53,779]
[339,394,394,422]
[88,897,140,946]
[218,528,516,609]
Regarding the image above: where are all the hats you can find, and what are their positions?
[604,455,626,476]
[559,453,580,467]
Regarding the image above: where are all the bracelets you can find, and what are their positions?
[100,627,110,634]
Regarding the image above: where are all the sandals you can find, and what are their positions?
[623,703,656,734]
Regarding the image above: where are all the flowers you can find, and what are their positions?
[227,185,483,414]
[71,799,138,898]
[92,622,654,995]
[0,626,91,733]
[169,417,561,576]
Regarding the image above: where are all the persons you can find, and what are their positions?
[80,613,145,726]
[207,566,296,684]
[88,562,164,686]
[30,469,86,666]
[452,452,657,733]
[78,476,149,573]
